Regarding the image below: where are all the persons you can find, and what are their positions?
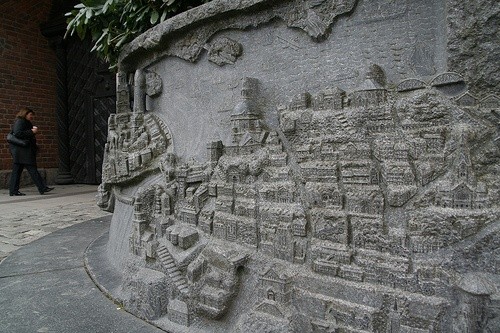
[9,108,55,196]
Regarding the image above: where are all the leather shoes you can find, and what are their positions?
[40,187,55,194]
[9,190,26,196]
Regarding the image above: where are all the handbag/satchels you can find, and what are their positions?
[7,119,28,147]
